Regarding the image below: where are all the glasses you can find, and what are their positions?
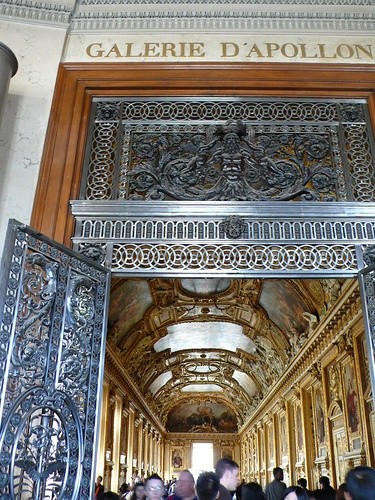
[148,487,164,492]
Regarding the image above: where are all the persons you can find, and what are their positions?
[94,458,375,500]
